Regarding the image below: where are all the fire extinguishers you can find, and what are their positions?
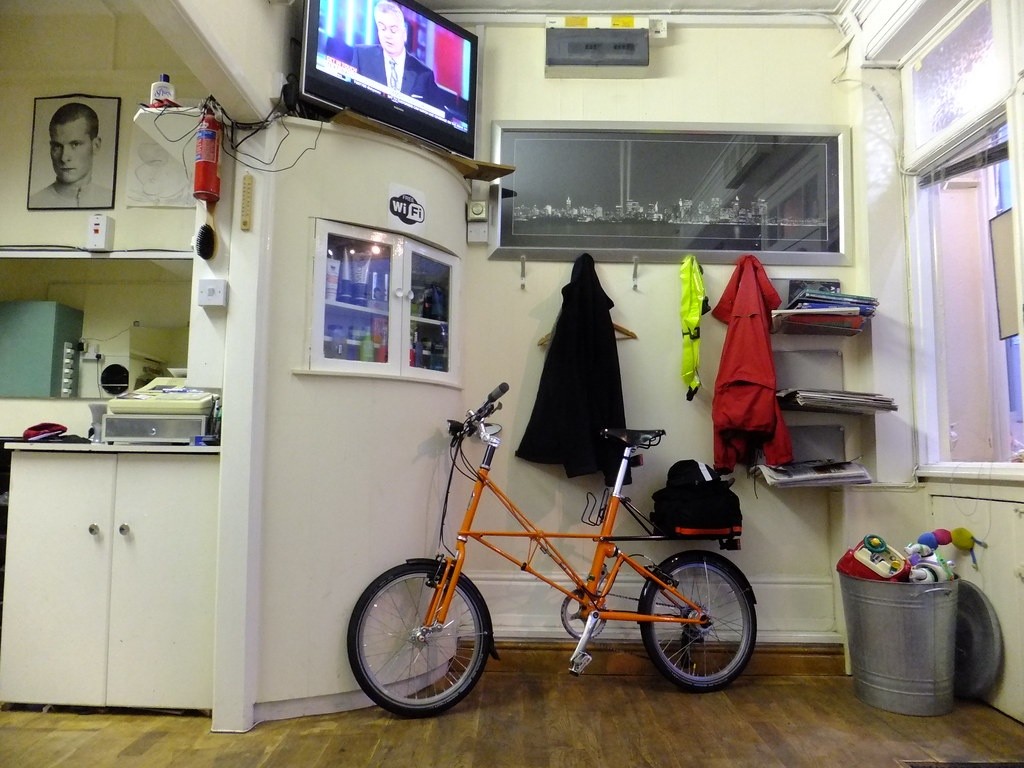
[193,103,223,201]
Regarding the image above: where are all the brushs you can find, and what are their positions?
[197,201,218,261]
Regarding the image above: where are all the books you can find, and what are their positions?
[746,460,872,487]
[775,288,879,329]
[779,389,896,416]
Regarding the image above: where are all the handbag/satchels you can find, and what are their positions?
[650,458,742,537]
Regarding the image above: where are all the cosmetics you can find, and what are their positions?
[326,243,444,373]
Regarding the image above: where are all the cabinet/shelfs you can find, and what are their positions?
[0,442,220,711]
[752,307,860,478]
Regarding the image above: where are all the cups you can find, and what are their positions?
[430,349,444,370]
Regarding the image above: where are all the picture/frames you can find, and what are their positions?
[26,93,122,210]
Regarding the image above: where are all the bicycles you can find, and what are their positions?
[347,382,758,719]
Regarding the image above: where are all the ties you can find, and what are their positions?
[388,58,399,88]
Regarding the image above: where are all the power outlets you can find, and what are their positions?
[79,344,103,359]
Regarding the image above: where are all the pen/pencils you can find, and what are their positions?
[205,395,222,435]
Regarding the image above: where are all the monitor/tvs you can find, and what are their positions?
[299,0,479,161]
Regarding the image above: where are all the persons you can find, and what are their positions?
[352,0,446,111]
[29,102,112,207]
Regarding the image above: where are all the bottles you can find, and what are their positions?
[409,332,424,366]
[328,318,387,362]
[150,74,174,105]
